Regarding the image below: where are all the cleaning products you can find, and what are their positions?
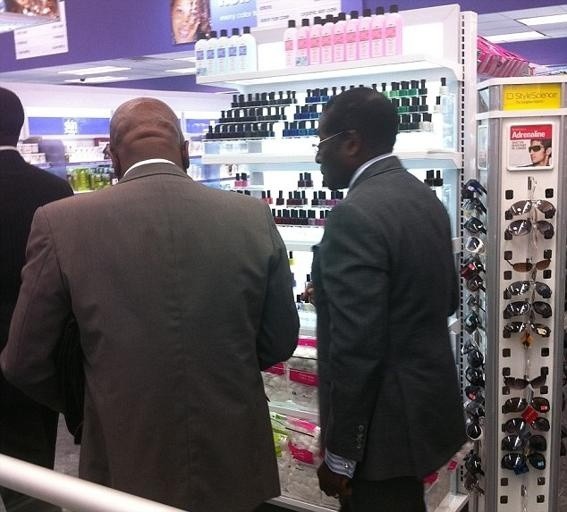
[284,7,403,67]
[194,26,257,79]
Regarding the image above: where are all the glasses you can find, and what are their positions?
[506,199,555,217]
[462,473,484,495]
[459,178,486,441]
[504,417,549,433]
[504,321,550,338]
[528,145,544,153]
[501,453,545,471]
[506,220,554,238]
[505,301,551,319]
[506,255,550,273]
[464,450,484,475]
[502,435,546,452]
[504,397,549,412]
[311,130,350,152]
[502,374,545,391]
[505,281,551,299]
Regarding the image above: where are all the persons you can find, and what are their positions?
[296,87,469,510]
[0,96,302,511]
[170,0,209,44]
[0,86,90,511]
[518,139,551,169]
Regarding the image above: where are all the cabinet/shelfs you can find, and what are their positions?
[195,3,478,507]
